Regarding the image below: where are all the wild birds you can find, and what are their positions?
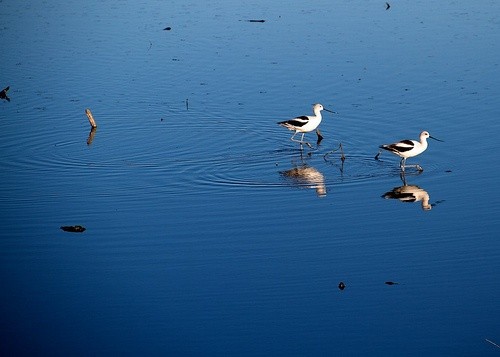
[382,178,449,216]
[278,99,340,156]
[378,129,445,177]
